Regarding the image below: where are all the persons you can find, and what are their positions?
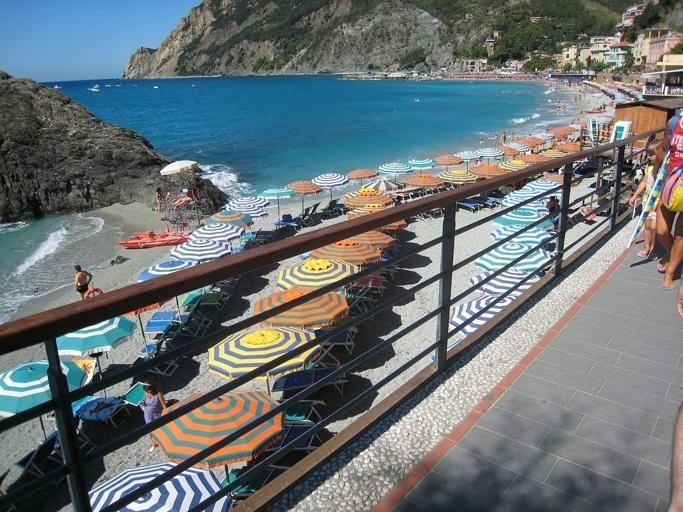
[137,378,167,453]
[75,265,92,300]
[547,196,560,225]
[154,187,166,214]
[665,272,683,511]
[554,74,641,115]
[628,112,683,290]
[480,128,550,145]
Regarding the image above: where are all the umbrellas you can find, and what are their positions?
[158,159,198,188]
[208,327,323,395]
[86,458,233,512]
[151,387,282,485]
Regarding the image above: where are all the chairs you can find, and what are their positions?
[411,198,495,221]
[0,196,398,510]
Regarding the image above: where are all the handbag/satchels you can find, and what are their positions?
[661,167,683,212]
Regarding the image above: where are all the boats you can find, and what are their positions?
[598,109,607,112]
[87,83,101,92]
[54,85,62,89]
[583,108,602,113]
[118,230,192,249]
